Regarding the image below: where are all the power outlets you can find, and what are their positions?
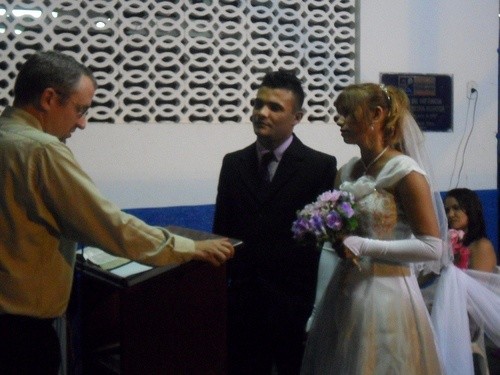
[467,81,476,100]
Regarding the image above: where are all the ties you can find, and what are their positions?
[256,150,279,216]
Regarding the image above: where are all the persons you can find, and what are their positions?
[301,84,443,375]
[444,188,497,273]
[0,51,234,375]
[212,71,337,375]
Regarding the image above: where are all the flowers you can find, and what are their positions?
[448,229,469,272]
[291,188,365,272]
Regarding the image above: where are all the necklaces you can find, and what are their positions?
[361,145,389,175]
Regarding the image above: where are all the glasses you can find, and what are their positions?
[56,90,88,118]
